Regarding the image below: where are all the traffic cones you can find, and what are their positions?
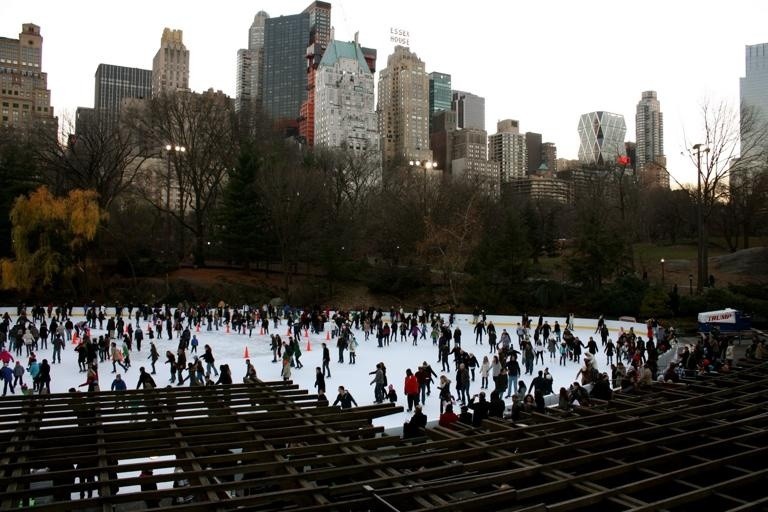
[244,345,250,358]
[286,327,330,352]
[124,323,129,332]
[259,327,265,336]
[195,322,200,332]
[146,323,151,331]
[118,349,124,361]
[71,333,77,344]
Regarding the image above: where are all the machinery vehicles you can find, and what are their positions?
[698,307,752,336]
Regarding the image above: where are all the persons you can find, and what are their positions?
[0,300,768,438]
[173,465,194,506]
[141,465,161,509]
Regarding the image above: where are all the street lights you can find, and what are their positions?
[688,274,693,295]
[166,144,186,264]
[660,258,665,286]
[408,159,438,283]
[693,143,702,293]
